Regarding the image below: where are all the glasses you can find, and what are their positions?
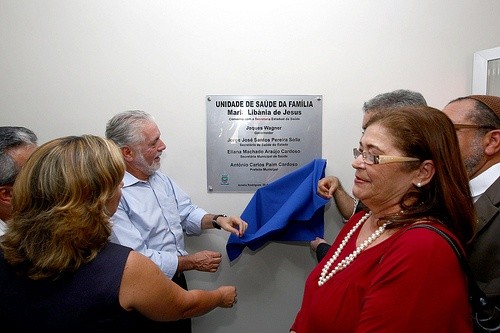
[353,147,420,166]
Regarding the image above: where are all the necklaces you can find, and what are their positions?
[318,209,409,286]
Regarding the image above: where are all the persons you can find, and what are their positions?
[105,111,248,332]
[0,134,238,333]
[309,91,426,261]
[0,127,38,235]
[441,94,499,333]
[288,106,477,333]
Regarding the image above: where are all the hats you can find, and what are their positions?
[465,95,500,121]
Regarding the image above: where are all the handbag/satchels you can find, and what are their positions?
[406,223,500,333]
